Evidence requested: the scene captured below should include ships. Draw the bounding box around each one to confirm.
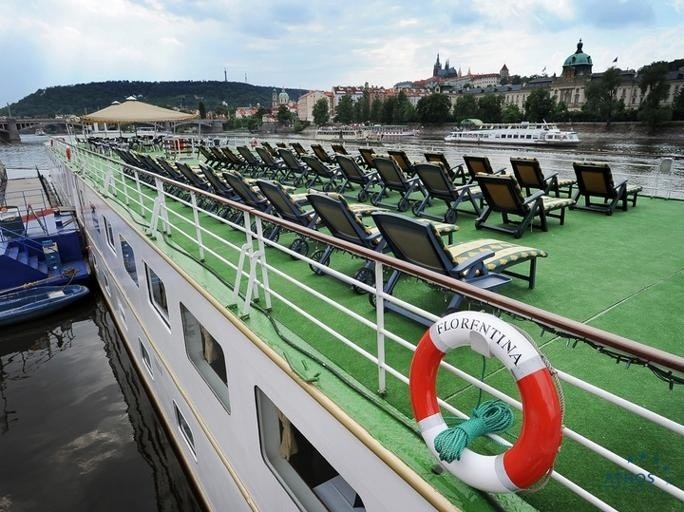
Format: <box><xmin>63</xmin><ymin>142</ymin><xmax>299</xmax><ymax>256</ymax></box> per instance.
<box><xmin>444</xmin><ymin>119</ymin><xmax>580</xmax><ymax>148</ymax></box>
<box><xmin>45</xmin><ymin>130</ymin><xmax>684</xmax><ymax>512</ymax></box>
<box><xmin>314</xmin><ymin>128</ymin><xmax>384</xmax><ymax>147</ymax></box>
<box><xmin>0</xmin><ymin>158</ymin><xmax>91</xmax><ymax>296</ymax></box>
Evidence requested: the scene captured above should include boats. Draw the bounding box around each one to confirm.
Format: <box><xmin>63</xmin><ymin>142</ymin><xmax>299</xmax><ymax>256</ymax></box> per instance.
<box><xmin>35</xmin><ymin>128</ymin><xmax>46</xmax><ymax>136</ymax></box>
<box><xmin>0</xmin><ymin>283</ymin><xmax>89</xmax><ymax>332</ymax></box>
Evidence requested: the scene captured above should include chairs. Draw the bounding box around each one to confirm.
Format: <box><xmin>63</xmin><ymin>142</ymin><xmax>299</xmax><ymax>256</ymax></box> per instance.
<box><xmin>300</xmin><ymin>153</ymin><xmax>355</xmax><ymax>192</ymax></box>
<box><xmin>358</xmin><ymin>148</ymin><xmax>376</xmax><ymax>171</ymax></box>
<box><xmin>113</xmin><ymin>148</ymin><xmax>202</xmax><ymax>191</ymax></box>
<box><xmin>306</xmin><ymin>188</ymin><xmax>459</xmax><ymax>295</ymax></box>
<box><xmin>254</xmin><ymin>179</ymin><xmax>391</xmax><ymax>261</ymax></box>
<box><xmin>209</xmin><ymin>146</ymin><xmax>237</xmax><ymax>170</ymax></box>
<box><xmin>568</xmin><ymin>162</ymin><xmax>643</xmax><ymax>216</ymax></box>
<box><xmin>195</xmin><ymin>146</ymin><xmax>223</xmax><ymax>167</ymax></box>
<box><xmin>331</xmin><ymin>144</ymin><xmax>365</xmax><ymax>169</ymax></box>
<box><xmin>221</xmin><ymin>168</ymin><xmax>312</xmax><ymax>240</ymax></box>
<box><xmin>510</xmin><ymin>157</ymin><xmax>576</xmax><ymax>198</ymax></box>
<box><xmin>236</xmin><ymin>145</ymin><xmax>276</xmax><ymax>178</ymax></box>
<box><xmin>198</xmin><ymin>161</ymin><xmax>297</xmax><ymax>223</ymax></box>
<box><xmin>74</xmin><ymin>133</ymin><xmax>230</xmax><ymax>160</ymax></box>
<box><xmin>311</xmin><ymin>144</ymin><xmax>338</xmax><ymax>166</ymax></box>
<box><xmin>292</xmin><ymin>143</ymin><xmax>314</xmax><ymax>159</ymax></box>
<box><xmin>412</xmin><ymin>161</ymin><xmax>488</xmax><ymax>224</ymax></box>
<box><xmin>261</xmin><ymin>141</ymin><xmax>277</xmax><ymax>156</ymax></box>
<box><xmin>255</xmin><ymin>147</ymin><xmax>296</xmax><ymax>180</ymax></box>
<box><xmin>220</xmin><ymin>147</ymin><xmax>255</xmax><ymax>171</ymax></box>
<box><xmin>463</xmin><ymin>154</ymin><xmax>507</xmax><ymax>181</ymax></box>
<box><xmin>370</xmin><ymin>154</ymin><xmax>433</xmax><ymax>212</ymax></box>
<box><xmin>424</xmin><ymin>152</ymin><xmax>474</xmax><ymax>186</ymax></box>
<box><xmin>155</xmin><ymin>156</ymin><xmax>222</xmax><ymax>202</ymax></box>
<box><xmin>278</xmin><ymin>149</ymin><xmax>324</xmax><ymax>188</ymax></box>
<box><xmin>387</xmin><ymin>150</ymin><xmax>419</xmax><ymax>178</ymax></box>
<box><xmin>335</xmin><ymin>156</ymin><xmax>390</xmax><ymax>202</ymax></box>
<box><xmin>369</xmin><ymin>212</ymin><xmax>548</xmax><ymax>318</ymax></box>
<box><xmin>175</xmin><ymin>162</ymin><xmax>257</xmax><ymax>212</ymax></box>
<box><xmin>475</xmin><ymin>172</ymin><xmax>577</xmax><ymax>239</ymax></box>
<box><xmin>276</xmin><ymin>143</ymin><xmax>286</xmax><ymax>160</ymax></box>
